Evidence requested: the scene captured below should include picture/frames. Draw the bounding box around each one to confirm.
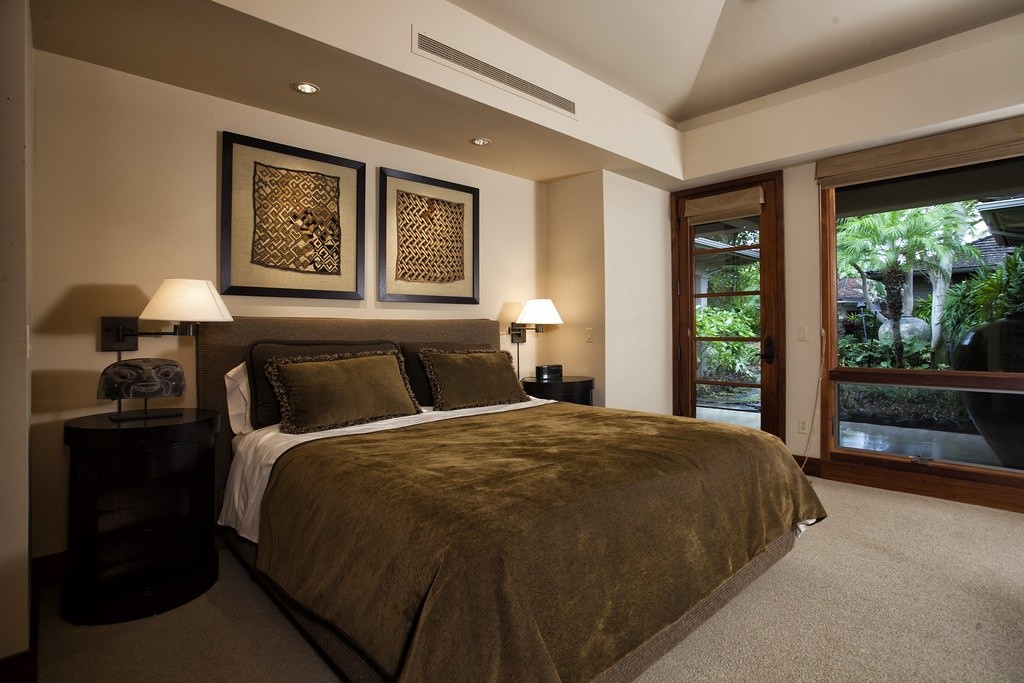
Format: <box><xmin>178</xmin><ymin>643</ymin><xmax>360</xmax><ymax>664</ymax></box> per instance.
<box><xmin>377</xmin><ymin>166</ymin><xmax>480</xmax><ymax>306</ymax></box>
<box><xmin>219</xmin><ymin>130</ymin><xmax>366</xmax><ymax>301</ymax></box>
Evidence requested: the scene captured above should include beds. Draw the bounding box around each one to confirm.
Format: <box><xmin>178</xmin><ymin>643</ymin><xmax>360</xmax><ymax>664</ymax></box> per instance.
<box><xmin>197</xmin><ymin>313</ymin><xmax>830</xmax><ymax>683</ymax></box>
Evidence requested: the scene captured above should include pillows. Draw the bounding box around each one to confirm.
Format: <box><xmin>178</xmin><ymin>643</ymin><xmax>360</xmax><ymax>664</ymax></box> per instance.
<box><xmin>396</xmin><ymin>340</ymin><xmax>498</xmax><ymax>407</ymax></box>
<box><xmin>416</xmin><ymin>346</ymin><xmax>532</xmax><ymax>412</ymax></box>
<box><xmin>264</xmin><ymin>348</ymin><xmax>423</xmax><ymax>435</ymax></box>
<box><xmin>245</xmin><ymin>337</ymin><xmax>401</xmax><ymax>431</ymax></box>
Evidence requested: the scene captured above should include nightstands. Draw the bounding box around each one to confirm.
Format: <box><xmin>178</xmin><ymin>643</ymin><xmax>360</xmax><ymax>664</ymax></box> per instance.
<box><xmin>60</xmin><ymin>409</ymin><xmax>223</xmax><ymax>627</ymax></box>
<box><xmin>522</xmin><ymin>376</ymin><xmax>595</xmax><ymax>406</ymax></box>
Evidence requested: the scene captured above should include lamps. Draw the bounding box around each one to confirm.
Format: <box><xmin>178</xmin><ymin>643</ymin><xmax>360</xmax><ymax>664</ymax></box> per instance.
<box><xmin>100</xmin><ymin>278</ymin><xmax>235</xmax><ymax>352</ymax></box>
<box><xmin>508</xmin><ymin>298</ymin><xmax>565</xmax><ymax>342</ymax></box>
